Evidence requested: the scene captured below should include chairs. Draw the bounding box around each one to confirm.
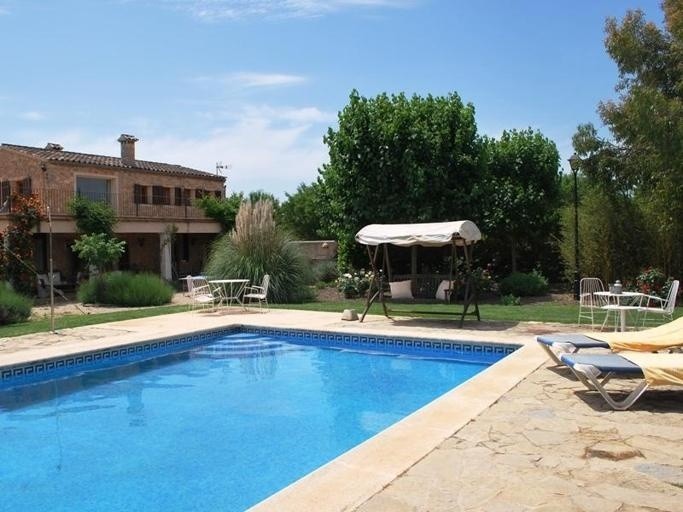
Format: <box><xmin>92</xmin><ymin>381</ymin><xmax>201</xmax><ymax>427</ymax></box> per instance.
<box><xmin>186</xmin><ymin>275</ymin><xmax>216</xmax><ymax>314</ymax></box>
<box><xmin>243</xmin><ymin>274</ymin><xmax>271</xmax><ymax>314</ymax></box>
<box><xmin>36</xmin><ymin>271</ymin><xmax>69</xmax><ymax>299</ymax></box>
<box><xmin>535</xmin><ymin>277</ymin><xmax>683</xmax><ymax>411</ymax></box>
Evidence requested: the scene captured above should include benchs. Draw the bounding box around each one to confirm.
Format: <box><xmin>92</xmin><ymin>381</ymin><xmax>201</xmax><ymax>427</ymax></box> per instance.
<box><xmin>360</xmin><ymin>273</ymin><xmax>480</xmax><ymax>329</ymax></box>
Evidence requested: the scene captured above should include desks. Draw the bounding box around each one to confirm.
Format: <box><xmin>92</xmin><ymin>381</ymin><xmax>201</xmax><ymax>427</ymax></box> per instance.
<box><xmin>207</xmin><ymin>278</ymin><xmax>250</xmax><ymax>312</ymax></box>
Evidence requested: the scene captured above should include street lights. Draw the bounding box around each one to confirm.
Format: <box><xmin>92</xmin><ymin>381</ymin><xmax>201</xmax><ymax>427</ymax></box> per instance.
<box><xmin>567</xmin><ymin>153</ymin><xmax>580</xmax><ymax>300</ymax></box>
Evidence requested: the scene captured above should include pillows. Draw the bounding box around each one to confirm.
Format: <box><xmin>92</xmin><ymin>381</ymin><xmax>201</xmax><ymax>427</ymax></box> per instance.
<box><xmin>435</xmin><ymin>279</ymin><xmax>455</xmax><ymax>302</ymax></box>
<box><xmin>388</xmin><ymin>279</ymin><xmax>415</xmax><ymax>300</ymax></box>
